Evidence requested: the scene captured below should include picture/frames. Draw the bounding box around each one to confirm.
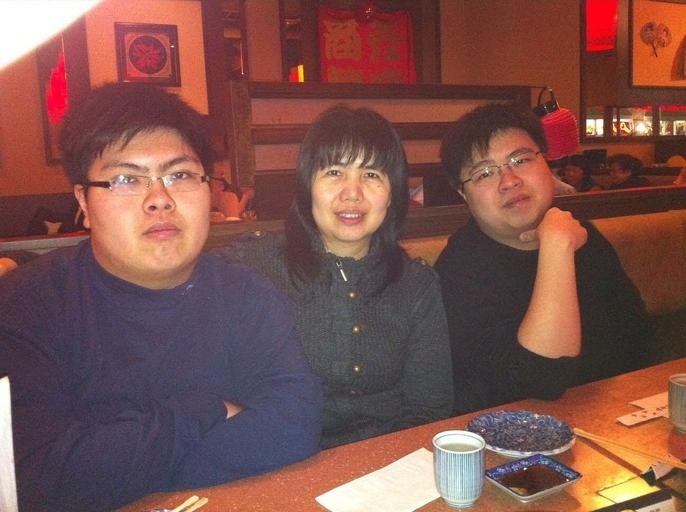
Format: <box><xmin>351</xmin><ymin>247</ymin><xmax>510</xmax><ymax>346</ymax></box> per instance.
<box><xmin>628</xmin><ymin>0</ymin><xmax>686</xmax><ymax>91</ymax></box>
<box><xmin>114</xmin><ymin>21</ymin><xmax>182</xmax><ymax>86</ymax></box>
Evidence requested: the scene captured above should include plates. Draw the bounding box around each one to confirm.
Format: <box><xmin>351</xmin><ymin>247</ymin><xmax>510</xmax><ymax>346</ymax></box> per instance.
<box><xmin>484</xmin><ymin>453</ymin><xmax>583</xmax><ymax>505</ymax></box>
<box><xmin>464</xmin><ymin>410</ymin><xmax>577</xmax><ymax>463</ymax></box>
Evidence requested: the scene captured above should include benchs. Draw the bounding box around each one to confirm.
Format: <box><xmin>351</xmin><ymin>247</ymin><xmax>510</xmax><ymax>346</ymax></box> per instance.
<box><xmin>589</xmin><ymin>166</ymin><xmax>681</xmax><ymax>189</ymax></box>
<box><xmin>15</xmin><ymin>185</ymin><xmax>685</xmax><ymax>365</ymax></box>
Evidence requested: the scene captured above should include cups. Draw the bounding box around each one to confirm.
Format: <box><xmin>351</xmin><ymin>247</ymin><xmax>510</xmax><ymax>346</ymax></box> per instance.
<box><xmin>668</xmin><ymin>373</ymin><xmax>686</xmax><ymax>437</ymax></box>
<box><xmin>432</xmin><ymin>430</ymin><xmax>485</xmax><ymax>509</ymax></box>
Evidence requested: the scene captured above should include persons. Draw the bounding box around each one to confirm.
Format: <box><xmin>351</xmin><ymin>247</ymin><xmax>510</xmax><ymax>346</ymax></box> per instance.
<box><xmin>1</xmin><ymin>80</ymin><xmax>324</xmax><ymax>512</ymax></box>
<box><xmin>556</xmin><ymin>153</ymin><xmax>603</xmax><ymax>194</ymax></box>
<box><xmin>433</xmin><ymin>102</ymin><xmax>656</xmax><ymax>417</ymax></box>
<box><xmin>222</xmin><ymin>100</ymin><xmax>456</xmax><ymax>451</ymax></box>
<box><xmin>607</xmin><ymin>154</ymin><xmax>651</xmax><ymax>190</ymax></box>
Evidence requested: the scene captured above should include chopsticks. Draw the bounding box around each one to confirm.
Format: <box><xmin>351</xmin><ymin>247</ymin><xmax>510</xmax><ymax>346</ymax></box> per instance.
<box><xmin>572</xmin><ymin>427</ymin><xmax>686</xmax><ymax>471</ymax></box>
<box><xmin>168</xmin><ymin>495</ymin><xmax>212</xmax><ymax>512</ymax></box>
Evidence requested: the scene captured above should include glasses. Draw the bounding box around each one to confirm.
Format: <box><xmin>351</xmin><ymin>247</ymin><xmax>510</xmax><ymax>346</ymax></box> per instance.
<box><xmin>84</xmin><ymin>172</ymin><xmax>209</xmax><ymax>196</ymax></box>
<box><xmin>461</xmin><ymin>149</ymin><xmax>542</xmax><ymax>192</ymax></box>
